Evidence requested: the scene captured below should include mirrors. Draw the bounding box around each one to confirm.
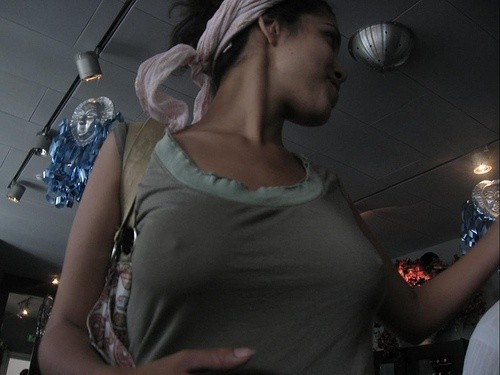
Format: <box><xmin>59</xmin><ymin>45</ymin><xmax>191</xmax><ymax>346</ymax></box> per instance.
<box><xmin>0</xmin><ymin>293</ymin><xmax>44</xmax><ymax>375</ymax></box>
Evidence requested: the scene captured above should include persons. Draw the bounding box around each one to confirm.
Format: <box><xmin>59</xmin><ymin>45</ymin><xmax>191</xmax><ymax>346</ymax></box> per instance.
<box><xmin>36</xmin><ymin>0</ymin><xmax>500</xmax><ymax>375</ymax></box>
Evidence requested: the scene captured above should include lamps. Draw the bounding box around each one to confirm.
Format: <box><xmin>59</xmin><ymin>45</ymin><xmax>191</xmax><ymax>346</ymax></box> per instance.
<box><xmin>471</xmin><ymin>145</ymin><xmax>493</xmax><ymax>175</ymax></box>
<box><xmin>16</xmin><ymin>305</ymin><xmax>29</xmax><ymax>320</ymax></box>
<box><xmin>31</xmin><ymin>135</ymin><xmax>51</xmax><ymax>156</ymax></box>
<box><xmin>7</xmin><ymin>184</ymin><xmax>26</xmax><ymax>203</ymax></box>
<box><xmin>73</xmin><ymin>51</ymin><xmax>102</xmax><ymax>81</ymax></box>
<box><xmin>346</xmin><ymin>20</ymin><xmax>417</xmax><ymax>72</ymax></box>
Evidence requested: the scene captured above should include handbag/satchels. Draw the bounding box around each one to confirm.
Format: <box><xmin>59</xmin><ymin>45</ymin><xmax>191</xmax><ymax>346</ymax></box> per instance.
<box><xmin>86</xmin><ymin>115</ymin><xmax>136</xmax><ymax>368</ymax></box>
